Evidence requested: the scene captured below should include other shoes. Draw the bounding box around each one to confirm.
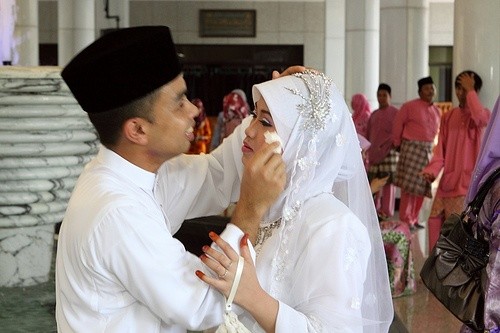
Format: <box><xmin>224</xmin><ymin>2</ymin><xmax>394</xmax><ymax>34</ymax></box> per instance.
<box><xmin>415</xmin><ymin>222</ymin><xmax>424</xmax><ymax>228</ymax></box>
<box><xmin>410</xmin><ymin>225</ymin><xmax>416</xmax><ymax>230</ymax></box>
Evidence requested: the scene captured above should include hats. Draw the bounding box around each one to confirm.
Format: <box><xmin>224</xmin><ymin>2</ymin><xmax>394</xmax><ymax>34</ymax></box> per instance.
<box><xmin>418</xmin><ymin>76</ymin><xmax>434</xmax><ymax>87</ymax></box>
<box><xmin>378</xmin><ymin>83</ymin><xmax>391</xmax><ymax>93</ymax></box>
<box><xmin>61</xmin><ymin>25</ymin><xmax>184</xmax><ymax>113</ymax></box>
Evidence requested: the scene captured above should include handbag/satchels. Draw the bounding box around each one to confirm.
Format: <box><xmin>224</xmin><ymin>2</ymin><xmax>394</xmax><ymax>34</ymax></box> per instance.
<box><xmin>214</xmin><ymin>256</ymin><xmax>252</xmax><ymax>333</ymax></box>
<box><xmin>420</xmin><ymin>167</ymin><xmax>500</xmax><ymax>333</ymax></box>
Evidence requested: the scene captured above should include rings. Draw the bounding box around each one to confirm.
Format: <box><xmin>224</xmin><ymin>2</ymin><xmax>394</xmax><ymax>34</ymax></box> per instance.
<box><xmin>219</xmin><ymin>270</ymin><xmax>227</xmax><ymax>279</ymax></box>
<box><xmin>228</xmin><ymin>262</ymin><xmax>233</xmax><ymax>270</ymax></box>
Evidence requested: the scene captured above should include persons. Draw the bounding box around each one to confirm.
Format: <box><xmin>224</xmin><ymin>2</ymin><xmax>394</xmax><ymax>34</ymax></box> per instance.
<box><xmin>362</xmin><ymin>83</ymin><xmax>403</xmax><ymax>225</ymax></box>
<box><xmin>55</xmin><ymin>25</ymin><xmax>308</xmax><ymax>333</ymax></box>
<box><xmin>459</xmin><ymin>95</ymin><xmax>500</xmax><ymax>333</ymax></box>
<box><xmin>349</xmin><ymin>94</ymin><xmax>371</xmax><ymax>173</ymax></box>
<box><xmin>422</xmin><ymin>70</ymin><xmax>491</xmax><ymax>256</ymax></box>
<box><xmin>392</xmin><ymin>76</ymin><xmax>442</xmax><ymax>232</ymax></box>
<box><xmin>195</xmin><ymin>69</ymin><xmax>395</xmax><ymax>333</ymax></box>
<box><xmin>186</xmin><ymin>89</ymin><xmax>250</xmax><ymax>156</ymax></box>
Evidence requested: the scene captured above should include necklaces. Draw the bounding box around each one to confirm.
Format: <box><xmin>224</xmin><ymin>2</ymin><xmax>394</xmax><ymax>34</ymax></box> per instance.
<box><xmin>252</xmin><ymin>216</ymin><xmax>282</xmax><ymax>247</ymax></box>
